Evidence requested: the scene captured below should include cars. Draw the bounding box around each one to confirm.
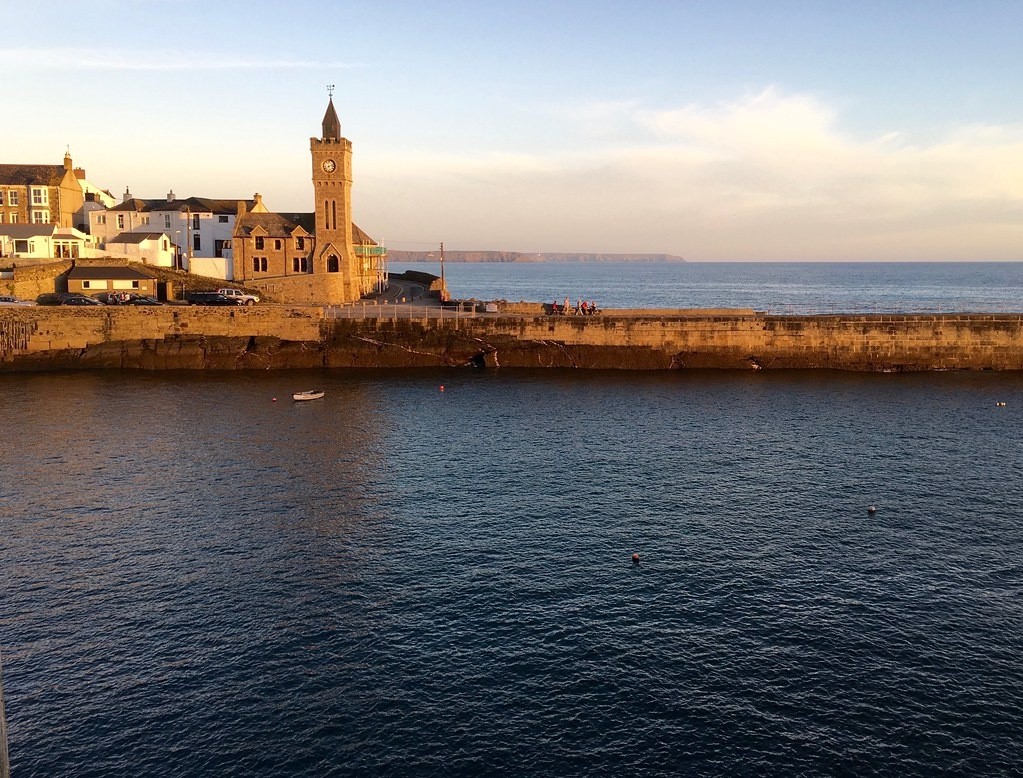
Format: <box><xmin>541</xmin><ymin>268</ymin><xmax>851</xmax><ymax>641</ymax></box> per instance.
<box><xmin>0</xmin><ymin>295</ymin><xmax>19</xmax><ymax>302</ymax></box>
<box><xmin>120</xmin><ymin>295</ymin><xmax>163</xmax><ymax>306</ymax></box>
<box><xmin>188</xmin><ymin>291</ymin><xmax>243</xmax><ymax>307</ymax></box>
<box><xmin>19</xmin><ymin>291</ymin><xmax>106</xmax><ymax>306</ymax></box>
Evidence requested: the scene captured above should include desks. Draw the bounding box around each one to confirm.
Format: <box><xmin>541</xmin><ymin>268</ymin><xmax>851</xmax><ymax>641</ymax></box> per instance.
<box><xmin>571</xmin><ymin>307</ymin><xmax>603</xmax><ymax>316</ymax></box>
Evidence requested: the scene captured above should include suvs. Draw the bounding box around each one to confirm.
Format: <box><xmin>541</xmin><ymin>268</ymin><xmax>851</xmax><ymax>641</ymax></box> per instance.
<box><xmin>218</xmin><ymin>288</ymin><xmax>260</xmax><ymax>306</ymax></box>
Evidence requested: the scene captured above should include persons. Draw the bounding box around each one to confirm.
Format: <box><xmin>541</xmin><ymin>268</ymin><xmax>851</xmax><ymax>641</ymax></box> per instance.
<box><xmin>218</xmin><ymin>290</ymin><xmax>224</xmax><ymax>297</ymax></box>
<box><xmin>552</xmin><ymin>301</ymin><xmax>559</xmax><ymax>315</ymax></box>
<box><xmin>121</xmin><ymin>291</ymin><xmax>125</xmax><ymax>300</ymax></box>
<box><xmin>125</xmin><ymin>293</ymin><xmax>130</xmax><ymax>301</ymax></box>
<box><xmin>105</xmin><ymin>292</ymin><xmax>110</xmax><ymax>304</ymax></box>
<box><xmin>131</xmin><ymin>291</ymin><xmax>138</xmax><ymax>300</ymax></box>
<box><xmin>563</xmin><ymin>297</ymin><xmax>570</xmax><ymax>316</ymax></box>
<box><xmin>589</xmin><ymin>301</ymin><xmax>597</xmax><ymax>315</ymax></box>
<box><xmin>112</xmin><ymin>292</ymin><xmax>121</xmax><ymax>305</ymax></box>
<box><xmin>581</xmin><ymin>300</ymin><xmax>590</xmax><ymax>315</ymax></box>
<box><xmin>576</xmin><ymin>297</ymin><xmax>584</xmax><ymax>316</ymax></box>
